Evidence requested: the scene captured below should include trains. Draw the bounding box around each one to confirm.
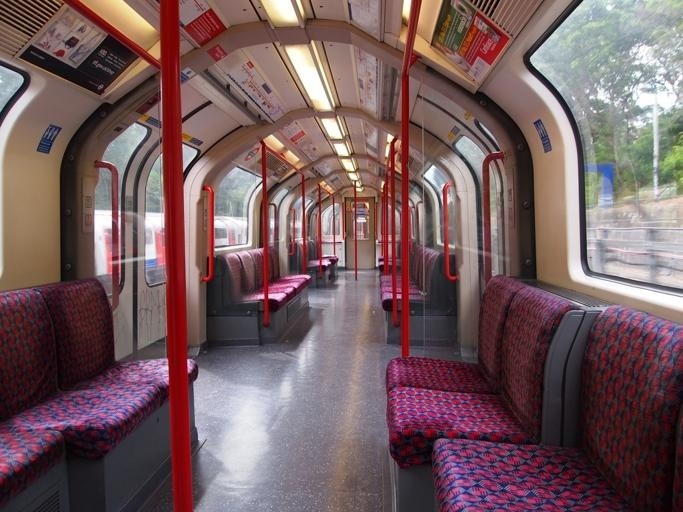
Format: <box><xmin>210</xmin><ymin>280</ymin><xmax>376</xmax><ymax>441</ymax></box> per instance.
<box><xmin>94</xmin><ymin>210</ymin><xmax>305</xmax><ymax>274</ymax></box>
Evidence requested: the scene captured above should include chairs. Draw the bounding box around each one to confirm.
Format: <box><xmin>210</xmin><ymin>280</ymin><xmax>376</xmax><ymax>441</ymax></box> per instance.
<box><xmin>0</xmin><ymin>276</ymin><xmax>201</xmax><ymax>511</ymax></box>
<box><xmin>377</xmin><ymin>237</ymin><xmax>682</xmax><ymax>510</ymax></box>
<box><xmin>202</xmin><ymin>237</ymin><xmax>340</xmax><ymax>348</ymax></box>
